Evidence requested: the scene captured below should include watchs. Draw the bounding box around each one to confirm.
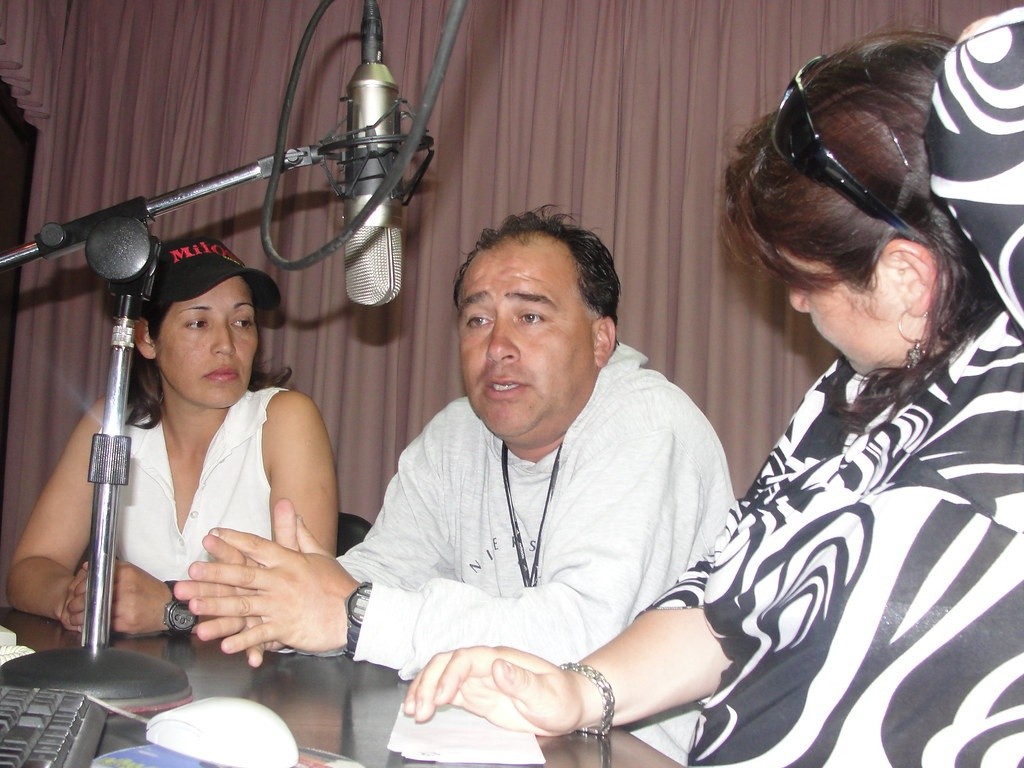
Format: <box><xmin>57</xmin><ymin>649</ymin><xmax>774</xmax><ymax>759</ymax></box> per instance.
<box><xmin>345</xmin><ymin>582</ymin><xmax>374</xmax><ymax>660</ymax></box>
<box><xmin>164</xmin><ymin>581</ymin><xmax>198</xmax><ymax>638</ymax></box>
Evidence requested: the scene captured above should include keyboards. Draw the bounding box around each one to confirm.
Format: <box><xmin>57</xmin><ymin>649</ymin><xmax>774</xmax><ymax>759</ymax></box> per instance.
<box><xmin>0</xmin><ymin>685</ymin><xmax>107</xmax><ymax>768</ymax></box>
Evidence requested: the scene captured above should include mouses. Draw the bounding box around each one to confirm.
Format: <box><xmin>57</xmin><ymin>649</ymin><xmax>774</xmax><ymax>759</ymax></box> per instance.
<box><xmin>145</xmin><ymin>697</ymin><xmax>300</xmax><ymax>768</ymax></box>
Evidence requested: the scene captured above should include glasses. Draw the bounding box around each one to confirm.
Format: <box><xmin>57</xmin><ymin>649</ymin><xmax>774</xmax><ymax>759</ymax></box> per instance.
<box><xmin>772</xmin><ymin>52</ymin><xmax>930</xmax><ymax>245</ymax></box>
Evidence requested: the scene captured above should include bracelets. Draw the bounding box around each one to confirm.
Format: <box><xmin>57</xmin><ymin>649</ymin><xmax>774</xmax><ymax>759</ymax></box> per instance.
<box><xmin>559</xmin><ymin>662</ymin><xmax>615</xmax><ymax>737</ymax></box>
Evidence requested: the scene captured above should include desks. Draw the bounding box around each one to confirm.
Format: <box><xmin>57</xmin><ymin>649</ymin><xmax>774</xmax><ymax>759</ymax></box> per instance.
<box><xmin>0</xmin><ymin>607</ymin><xmax>687</xmax><ymax>768</ymax></box>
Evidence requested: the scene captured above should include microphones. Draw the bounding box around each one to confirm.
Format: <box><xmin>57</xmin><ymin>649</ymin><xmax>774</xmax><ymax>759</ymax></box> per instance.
<box><xmin>345</xmin><ymin>0</ymin><xmax>402</xmax><ymax>307</ymax></box>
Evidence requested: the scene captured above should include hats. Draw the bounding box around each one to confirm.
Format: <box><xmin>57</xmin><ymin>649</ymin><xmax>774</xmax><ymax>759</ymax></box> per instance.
<box><xmin>147</xmin><ymin>236</ymin><xmax>281</xmax><ymax>311</ymax></box>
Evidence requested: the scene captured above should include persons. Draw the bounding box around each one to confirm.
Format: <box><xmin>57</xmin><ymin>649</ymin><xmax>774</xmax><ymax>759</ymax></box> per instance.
<box><xmin>6</xmin><ymin>232</ymin><xmax>339</xmax><ymax>636</ymax></box>
<box><xmin>174</xmin><ymin>202</ymin><xmax>738</xmax><ymax>767</ymax></box>
<box><xmin>405</xmin><ymin>5</ymin><xmax>1024</xmax><ymax>768</ymax></box>
